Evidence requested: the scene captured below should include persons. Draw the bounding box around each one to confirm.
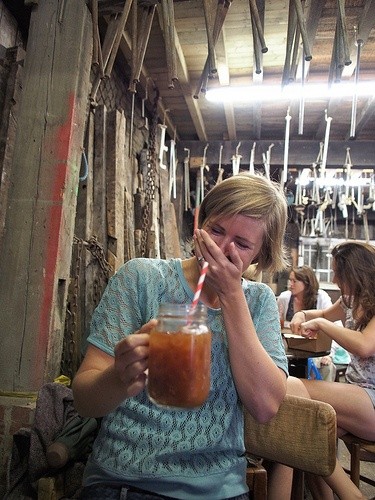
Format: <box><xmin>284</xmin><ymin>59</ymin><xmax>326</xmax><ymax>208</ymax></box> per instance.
<box><xmin>70</xmin><ymin>171</ymin><xmax>290</xmax><ymax>500</ymax></box>
<box><xmin>269</xmin><ymin>240</ymin><xmax>375</xmax><ymax>500</ymax></box>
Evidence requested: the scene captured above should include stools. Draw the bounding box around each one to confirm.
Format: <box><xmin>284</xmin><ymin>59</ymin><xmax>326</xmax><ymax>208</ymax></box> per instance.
<box><xmin>336</xmin><ymin>432</ymin><xmax>375</xmax><ymax>500</ymax></box>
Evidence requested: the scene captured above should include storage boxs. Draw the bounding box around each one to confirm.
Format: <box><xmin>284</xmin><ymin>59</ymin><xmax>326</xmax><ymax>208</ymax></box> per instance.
<box><xmin>284</xmin><ymin>331</ymin><xmax>332</xmax><ymax>353</ymax></box>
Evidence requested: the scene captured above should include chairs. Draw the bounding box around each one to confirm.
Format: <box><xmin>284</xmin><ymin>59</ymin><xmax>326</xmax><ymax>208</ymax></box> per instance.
<box><xmin>243</xmin><ymin>395</ymin><xmax>337</xmax><ymax>500</ymax></box>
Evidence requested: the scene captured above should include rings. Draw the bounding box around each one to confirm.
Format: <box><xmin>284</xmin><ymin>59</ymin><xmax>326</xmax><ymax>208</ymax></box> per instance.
<box><xmin>196</xmin><ymin>255</ymin><xmax>205</xmax><ymax>263</ymax></box>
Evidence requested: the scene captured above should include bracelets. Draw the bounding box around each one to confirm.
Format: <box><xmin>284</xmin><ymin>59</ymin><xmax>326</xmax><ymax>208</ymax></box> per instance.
<box><xmin>301</xmin><ymin>310</ymin><xmax>306</xmax><ymax>316</ymax></box>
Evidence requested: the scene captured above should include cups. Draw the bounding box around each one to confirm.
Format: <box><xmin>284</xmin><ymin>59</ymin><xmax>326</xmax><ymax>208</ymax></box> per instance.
<box><xmin>138</xmin><ymin>303</ymin><xmax>211</xmax><ymax>409</ymax></box>
